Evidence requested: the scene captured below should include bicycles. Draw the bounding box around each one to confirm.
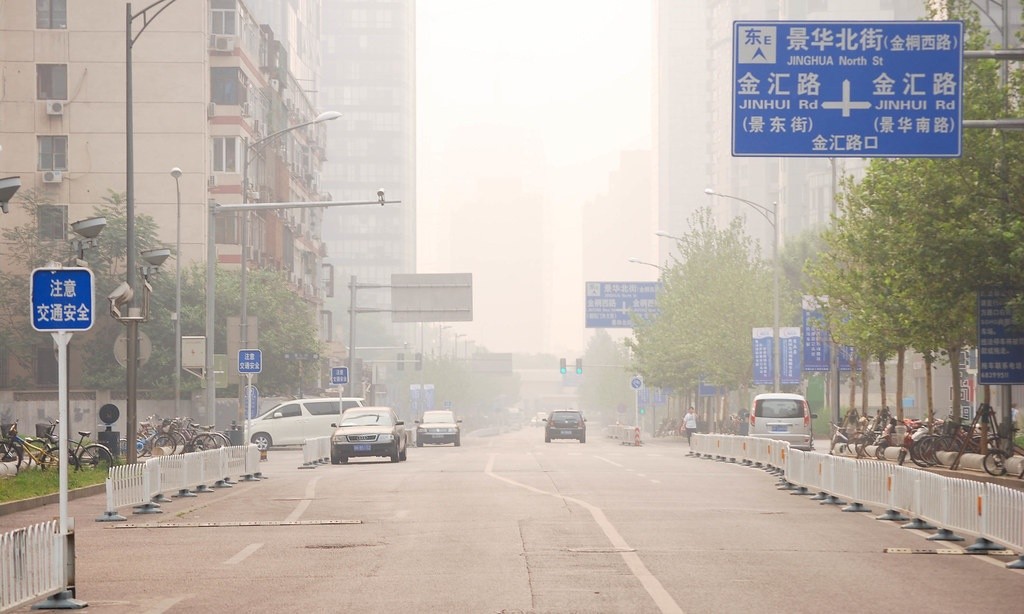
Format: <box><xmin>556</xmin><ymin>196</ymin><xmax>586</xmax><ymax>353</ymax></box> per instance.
<box><xmin>120</xmin><ymin>414</ymin><xmax>234</xmax><ymax>459</ymax></box>
<box><xmin>828</xmin><ymin>414</ymin><xmax>1024</xmax><ymax>477</ymax></box>
<box><xmin>655</xmin><ymin>413</ymin><xmax>741</xmax><ymax>437</ymax></box>
<box><xmin>0</xmin><ymin>418</ymin><xmax>114</xmax><ymax>475</ymax></box>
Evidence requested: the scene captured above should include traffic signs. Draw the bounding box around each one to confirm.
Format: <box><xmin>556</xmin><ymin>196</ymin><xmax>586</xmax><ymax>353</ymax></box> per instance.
<box><xmin>584</xmin><ymin>281</ymin><xmax>666</xmax><ymax>329</ymax></box>
<box><xmin>732</xmin><ymin>20</ymin><xmax>963</xmax><ymax>159</ymax></box>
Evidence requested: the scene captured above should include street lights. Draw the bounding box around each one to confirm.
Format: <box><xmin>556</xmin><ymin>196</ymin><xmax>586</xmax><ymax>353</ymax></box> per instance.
<box><xmin>439</xmin><ymin>325</ymin><xmax>452</xmax><ymax>358</ymax></box>
<box><xmin>704</xmin><ymin>189</ymin><xmax>780</xmax><ymax>394</ymax></box>
<box><xmin>455</xmin><ymin>333</ymin><xmax>475</xmax><ymax>359</ymax></box>
<box><xmin>172</xmin><ymin>167</ymin><xmax>184</xmax><ymax>420</ymax></box>
<box><xmin>238</xmin><ymin>111</ymin><xmax>342</xmax><ymax>415</ymax></box>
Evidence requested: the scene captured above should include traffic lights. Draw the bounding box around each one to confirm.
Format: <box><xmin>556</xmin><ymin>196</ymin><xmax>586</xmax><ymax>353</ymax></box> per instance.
<box><xmin>560</xmin><ymin>358</ymin><xmax>567</xmax><ymax>374</ymax></box>
<box><xmin>576</xmin><ymin>358</ymin><xmax>582</xmax><ymax>374</ymax></box>
<box><xmin>640</xmin><ymin>408</ymin><xmax>645</xmax><ymax>415</ymax></box>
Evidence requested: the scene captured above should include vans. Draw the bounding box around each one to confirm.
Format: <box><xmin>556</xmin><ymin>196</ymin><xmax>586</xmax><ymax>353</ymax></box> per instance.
<box><xmin>743</xmin><ymin>393</ymin><xmax>818</xmax><ymax>452</ymax></box>
<box><xmin>243</xmin><ymin>398</ymin><xmax>367</xmax><ymax>452</ymax></box>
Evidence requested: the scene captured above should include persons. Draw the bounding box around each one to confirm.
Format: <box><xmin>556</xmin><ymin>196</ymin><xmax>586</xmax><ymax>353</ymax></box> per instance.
<box><xmin>1011</xmin><ymin>402</ymin><xmax>1018</xmax><ymax>428</ymax></box>
<box><xmin>684</xmin><ymin>407</ymin><xmax>696</xmax><ymax>445</ymax></box>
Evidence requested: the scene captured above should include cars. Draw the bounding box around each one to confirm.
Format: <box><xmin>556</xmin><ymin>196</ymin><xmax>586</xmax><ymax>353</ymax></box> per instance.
<box><xmin>415</xmin><ymin>410</ymin><xmax>463</xmax><ymax>447</ymax></box>
<box><xmin>530</xmin><ymin>412</ymin><xmax>549</xmax><ymax>427</ymax></box>
<box><xmin>330</xmin><ymin>406</ymin><xmax>408</xmax><ymax>465</ymax></box>
<box><xmin>542</xmin><ymin>410</ymin><xmax>587</xmax><ymax>443</ymax></box>
